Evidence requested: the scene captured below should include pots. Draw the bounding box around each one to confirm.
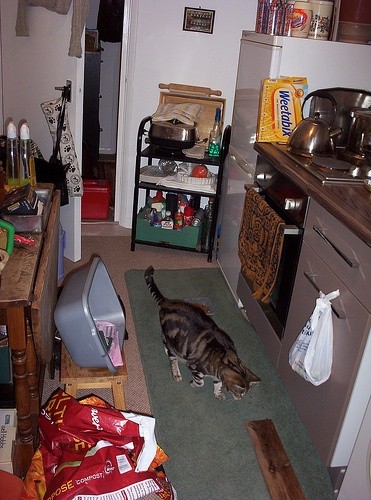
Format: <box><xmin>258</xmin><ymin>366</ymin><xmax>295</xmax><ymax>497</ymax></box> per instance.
<box><xmin>349</xmin><ymin>108</ymin><xmax>371</xmax><ymax>156</ymax></box>
<box><xmin>142</xmin><ymin>118</ymin><xmax>209</xmax><ymax>155</ymax></box>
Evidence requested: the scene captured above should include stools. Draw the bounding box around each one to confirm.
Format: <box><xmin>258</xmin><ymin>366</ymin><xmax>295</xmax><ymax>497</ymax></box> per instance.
<box><xmin>60</xmin><ymin>339</ymin><xmax>129</xmax><ymax>413</ymax></box>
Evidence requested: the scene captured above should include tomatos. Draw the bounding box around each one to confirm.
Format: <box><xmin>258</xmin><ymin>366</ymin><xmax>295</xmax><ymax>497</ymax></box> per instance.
<box><xmin>193</xmin><ymin>165</ymin><xmax>208</xmax><ymax>179</ymax></box>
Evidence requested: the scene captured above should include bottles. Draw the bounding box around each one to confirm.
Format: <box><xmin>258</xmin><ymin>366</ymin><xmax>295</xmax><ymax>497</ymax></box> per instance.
<box><xmin>19</xmin><ymin>123</ymin><xmax>37</xmax><ymax>186</ymax></box>
<box><xmin>208</xmin><ymin>108</ymin><xmax>222</xmax><ymax>158</ymax></box>
<box><xmin>161</xmin><ymin>192</ymin><xmax>204</xmax><ymax>230</ymax></box>
<box><xmin>6</xmin><ymin>121</ymin><xmax>19</xmax><ymax>186</ymax></box>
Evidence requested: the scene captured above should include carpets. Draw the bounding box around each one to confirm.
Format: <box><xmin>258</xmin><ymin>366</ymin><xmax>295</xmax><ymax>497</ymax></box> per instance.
<box><xmin>125</xmin><ymin>267</ymin><xmax>336</xmax><ymax>500</ymax></box>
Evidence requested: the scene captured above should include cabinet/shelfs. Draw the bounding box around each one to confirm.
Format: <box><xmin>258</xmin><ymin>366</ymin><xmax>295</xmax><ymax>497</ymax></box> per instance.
<box><xmin>276</xmin><ymin>198</ymin><xmax>371</xmax><ymax>498</ymax></box>
<box><xmin>130</xmin><ymin>116</ymin><xmax>232</xmax><ymax>263</ymax></box>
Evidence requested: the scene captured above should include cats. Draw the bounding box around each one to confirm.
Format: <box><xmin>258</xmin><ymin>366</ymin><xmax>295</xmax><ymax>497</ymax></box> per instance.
<box><xmin>144</xmin><ymin>265</ymin><xmax>262</xmax><ymax>401</ymax></box>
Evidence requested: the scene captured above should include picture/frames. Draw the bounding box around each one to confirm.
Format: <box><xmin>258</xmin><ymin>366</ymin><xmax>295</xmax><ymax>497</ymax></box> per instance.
<box><xmin>183</xmin><ymin>7</ymin><xmax>215</xmax><ymax>34</ymax></box>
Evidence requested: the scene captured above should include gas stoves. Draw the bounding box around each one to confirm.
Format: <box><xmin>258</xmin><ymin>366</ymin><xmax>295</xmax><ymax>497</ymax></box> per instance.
<box><xmin>271</xmin><ymin>140</ymin><xmax>371</xmax><ymax>187</ymax></box>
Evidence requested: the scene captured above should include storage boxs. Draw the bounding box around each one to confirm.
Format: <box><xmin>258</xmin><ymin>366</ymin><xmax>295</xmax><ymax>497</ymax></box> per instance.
<box><xmin>135</xmin><ymin>202</ymin><xmax>204</xmax><ymax>248</ymax></box>
<box><xmin>256</xmin><ymin>76</ymin><xmax>308</xmax><ymax>143</ymax></box>
<box><xmin>54</xmin><ymin>256</ymin><xmax>125</xmax><ymax>373</ymax></box>
<box><xmin>0</xmin><ymin>334</ymin><xmax>14</xmax><ymax>385</ymax></box>
<box><xmin>82</xmin><ymin>180</ymin><xmax>112</xmax><ymax>219</ymax></box>
<box><xmin>0</xmin><ymin>409</ymin><xmax>17</xmax><ymax>475</ymax></box>
<box><xmin>0</xmin><ymin>183</ymin><xmax>51</xmax><ymax>233</ymax></box>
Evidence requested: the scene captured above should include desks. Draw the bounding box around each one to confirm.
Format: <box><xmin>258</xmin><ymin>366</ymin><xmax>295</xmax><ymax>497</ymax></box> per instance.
<box><xmin>0</xmin><ymin>182</ymin><xmax>61</xmax><ymax>479</ymax></box>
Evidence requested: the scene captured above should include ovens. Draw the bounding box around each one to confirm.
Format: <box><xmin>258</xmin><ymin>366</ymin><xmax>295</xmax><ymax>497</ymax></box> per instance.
<box><xmin>240</xmin><ymin>155</ymin><xmax>311</xmax><ymax>342</ymax></box>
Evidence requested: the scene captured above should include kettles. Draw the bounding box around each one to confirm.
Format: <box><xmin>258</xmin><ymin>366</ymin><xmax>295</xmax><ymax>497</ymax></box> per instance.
<box><xmin>287</xmin><ymin>92</ymin><xmax>343</xmax><ymax>158</ymax></box>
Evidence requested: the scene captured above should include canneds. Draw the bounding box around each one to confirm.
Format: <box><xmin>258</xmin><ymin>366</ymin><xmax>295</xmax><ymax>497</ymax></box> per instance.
<box><xmin>290</xmin><ymin>1</ymin><xmax>313</xmax><ymax>38</ymax></box>
